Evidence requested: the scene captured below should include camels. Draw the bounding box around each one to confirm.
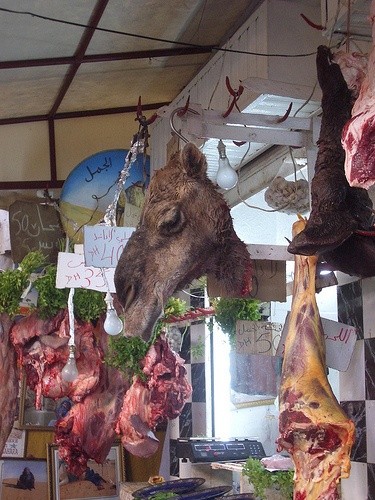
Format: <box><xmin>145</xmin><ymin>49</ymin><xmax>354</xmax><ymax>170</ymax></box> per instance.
<box><xmin>112</xmin><ymin>143</ymin><xmax>252</xmax><ymax>342</ymax></box>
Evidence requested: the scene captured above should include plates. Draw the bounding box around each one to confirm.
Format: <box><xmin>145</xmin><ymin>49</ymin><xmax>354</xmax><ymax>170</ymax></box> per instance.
<box><xmin>131</xmin><ymin>478</ymin><xmax>205</xmax><ymax>500</ymax></box>
<box><xmin>178</xmin><ymin>486</ymin><xmax>233</xmax><ymax>500</ymax></box>
<box><xmin>207</xmin><ymin>493</ymin><xmax>259</xmax><ymax>500</ymax></box>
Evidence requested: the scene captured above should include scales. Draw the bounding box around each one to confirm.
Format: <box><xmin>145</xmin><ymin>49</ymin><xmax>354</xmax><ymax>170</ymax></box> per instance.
<box><xmin>170</xmin><ymin>436</ymin><xmax>267</xmax><ymax>463</ymax></box>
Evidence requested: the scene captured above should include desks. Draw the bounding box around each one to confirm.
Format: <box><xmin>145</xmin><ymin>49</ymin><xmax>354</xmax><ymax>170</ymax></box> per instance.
<box><xmin>120</xmin><ymin>479</ymin><xmax>179</xmax><ymax>500</ymax></box>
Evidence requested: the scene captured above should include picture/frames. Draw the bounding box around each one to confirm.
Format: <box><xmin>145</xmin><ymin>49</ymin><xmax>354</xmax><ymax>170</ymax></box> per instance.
<box><xmin>45</xmin><ymin>438</ymin><xmax>125</xmax><ymax>500</ymax></box>
